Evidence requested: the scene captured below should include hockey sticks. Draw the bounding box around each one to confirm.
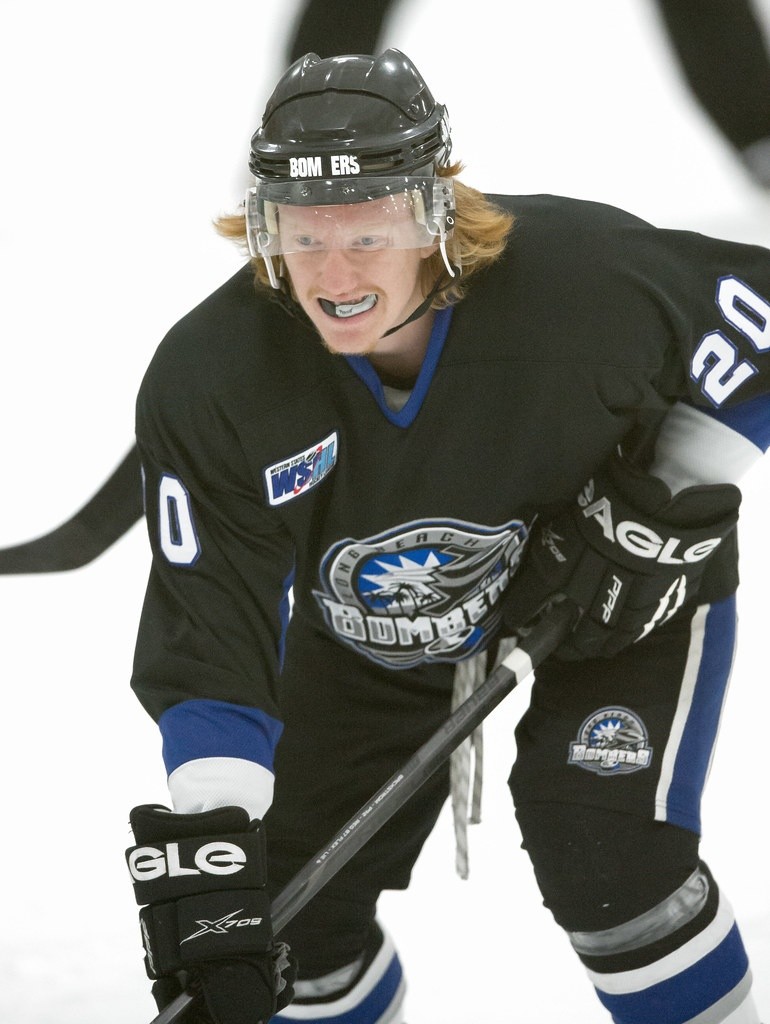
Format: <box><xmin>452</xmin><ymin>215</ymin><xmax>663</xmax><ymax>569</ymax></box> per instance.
<box><xmin>143</xmin><ymin>597</ymin><xmax>585</xmax><ymax>1024</ymax></box>
<box><xmin>0</xmin><ymin>441</ymin><xmax>146</xmax><ymax>577</ymax></box>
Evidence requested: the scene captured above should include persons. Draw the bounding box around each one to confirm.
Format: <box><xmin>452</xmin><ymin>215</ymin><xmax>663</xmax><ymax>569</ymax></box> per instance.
<box><xmin>123</xmin><ymin>48</ymin><xmax>768</xmax><ymax>1024</ymax></box>
<box><xmin>289</xmin><ymin>0</ymin><xmax>770</xmax><ymax>193</ymax></box>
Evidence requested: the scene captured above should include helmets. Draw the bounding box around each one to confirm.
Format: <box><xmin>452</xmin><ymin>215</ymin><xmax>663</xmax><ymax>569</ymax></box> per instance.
<box><xmin>243</xmin><ymin>47</ymin><xmax>455</xmax><ymax>290</ymax></box>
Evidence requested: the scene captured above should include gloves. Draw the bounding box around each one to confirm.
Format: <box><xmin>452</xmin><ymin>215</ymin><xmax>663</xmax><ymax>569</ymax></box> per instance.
<box><xmin>125</xmin><ymin>804</ymin><xmax>298</xmax><ymax>1024</ymax></box>
<box><xmin>497</xmin><ymin>455</ymin><xmax>742</xmax><ymax>669</ymax></box>
